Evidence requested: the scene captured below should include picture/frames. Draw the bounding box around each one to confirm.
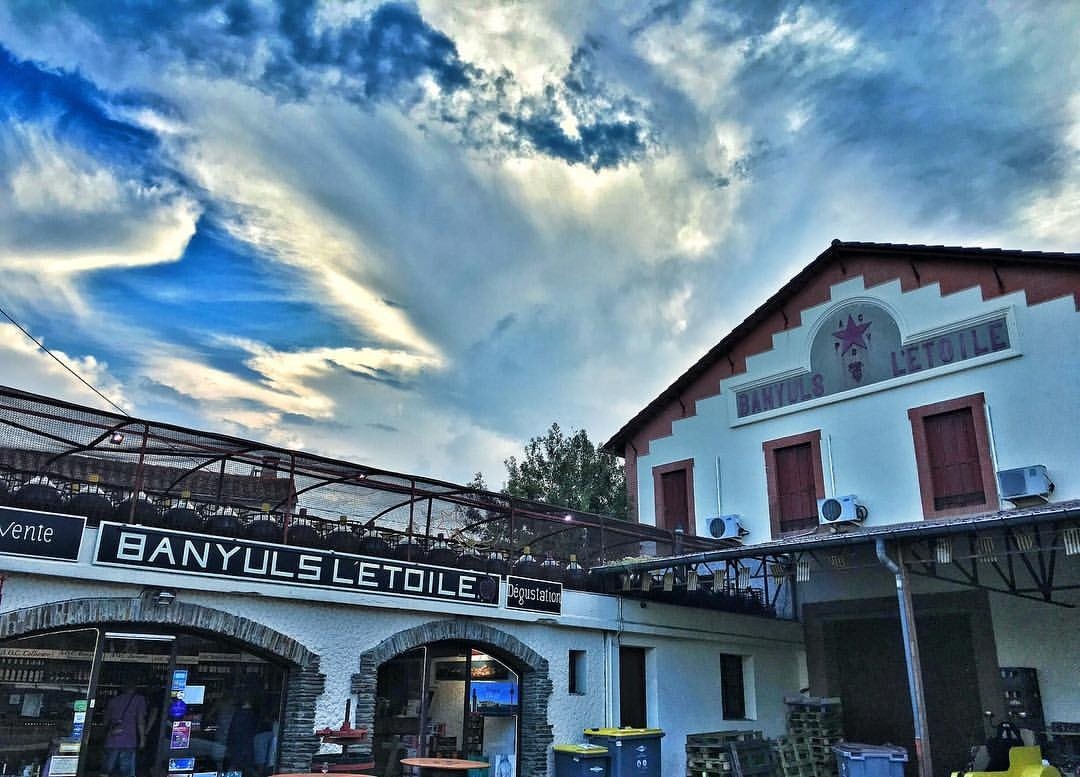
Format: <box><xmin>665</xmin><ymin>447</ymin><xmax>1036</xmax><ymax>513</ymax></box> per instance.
<box><xmin>436</xmin><ymin>659</ymin><xmax>510</xmax><ymax>680</ymax></box>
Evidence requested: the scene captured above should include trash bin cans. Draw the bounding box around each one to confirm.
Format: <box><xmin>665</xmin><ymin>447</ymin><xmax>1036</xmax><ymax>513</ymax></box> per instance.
<box><xmin>831</xmin><ymin>742</ymin><xmax>909</xmax><ymax>777</ymax></box>
<box><xmin>553</xmin><ymin>743</ymin><xmax>609</xmax><ymax>777</ymax></box>
<box><xmin>583</xmin><ymin>725</ymin><xmax>666</xmax><ymax>777</ymax></box>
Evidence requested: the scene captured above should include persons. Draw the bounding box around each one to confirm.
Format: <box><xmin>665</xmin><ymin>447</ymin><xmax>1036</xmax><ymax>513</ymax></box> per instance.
<box><xmin>141</xmin><ymin>686</ymin><xmax>166</xmax><ymax>777</ymax></box>
<box><xmin>99</xmin><ymin>677</ymin><xmax>147</xmax><ymax>777</ymax></box>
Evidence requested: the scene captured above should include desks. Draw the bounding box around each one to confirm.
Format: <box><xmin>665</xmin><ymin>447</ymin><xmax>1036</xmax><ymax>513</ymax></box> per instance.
<box><xmin>401</xmin><ymin>757</ymin><xmax>490</xmax><ymax>777</ymax></box>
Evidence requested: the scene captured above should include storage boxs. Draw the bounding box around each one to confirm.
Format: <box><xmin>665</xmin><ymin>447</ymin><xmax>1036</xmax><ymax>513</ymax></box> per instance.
<box><xmin>999</xmin><ymin>666</ymin><xmax>1080</xmax><ymax>768</ymax></box>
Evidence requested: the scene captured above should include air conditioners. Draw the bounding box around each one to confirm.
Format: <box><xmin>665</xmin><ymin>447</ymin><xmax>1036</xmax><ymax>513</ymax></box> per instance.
<box><xmin>706</xmin><ymin>515</ymin><xmax>742</xmax><ymax>539</ymax></box>
<box><xmin>817</xmin><ymin>495</ymin><xmax>859</xmax><ymax>523</ymax></box>
<box><xmin>996</xmin><ymin>465</ymin><xmax>1049</xmax><ymax>496</ymax></box>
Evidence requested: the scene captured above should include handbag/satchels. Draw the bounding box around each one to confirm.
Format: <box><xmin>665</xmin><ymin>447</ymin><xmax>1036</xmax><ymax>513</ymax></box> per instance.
<box><xmin>109</xmin><ymin>719</ymin><xmax>123</xmax><ymax>736</ymax></box>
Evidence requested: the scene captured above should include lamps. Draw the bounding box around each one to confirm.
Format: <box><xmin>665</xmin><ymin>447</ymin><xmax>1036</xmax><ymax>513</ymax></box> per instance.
<box><xmin>104</xmin><ymin>632</ymin><xmax>176</xmax><ymax>642</ymax></box>
<box><xmin>154</xmin><ymin>589</ymin><xmax>176</xmax><ymax>608</ymax></box>
